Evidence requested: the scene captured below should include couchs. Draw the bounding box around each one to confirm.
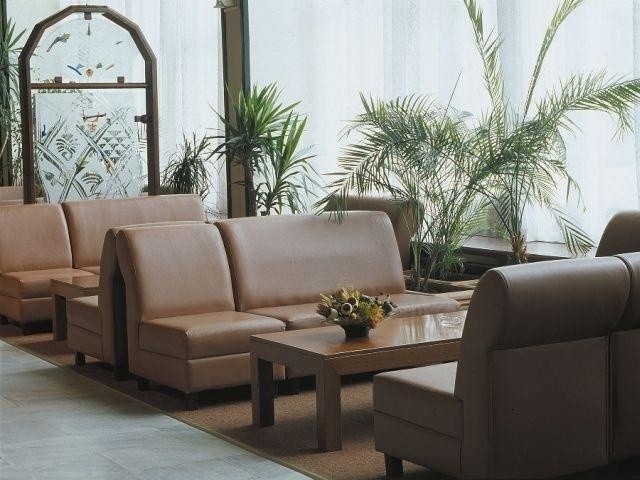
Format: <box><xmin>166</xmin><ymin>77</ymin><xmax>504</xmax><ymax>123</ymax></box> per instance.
<box><xmin>368</xmin><ymin>207</ymin><xmax>640</xmax><ymax>480</ymax></box>
<box><xmin>328</xmin><ymin>195</ymin><xmax>425</xmax><ymax>290</ymax></box>
<box><xmin>115</xmin><ymin>210</ymin><xmax>462</xmax><ymax>411</ymax></box>
<box><xmin>2</xmin><ymin>185</ymin><xmax>43</xmax><ymax>207</ymax></box>
<box><xmin>67</xmin><ymin>219</ymin><xmax>214</xmax><ymax>373</ymax></box>
<box><xmin>4</xmin><ymin>194</ymin><xmax>208</xmax><ymax>336</ymax></box>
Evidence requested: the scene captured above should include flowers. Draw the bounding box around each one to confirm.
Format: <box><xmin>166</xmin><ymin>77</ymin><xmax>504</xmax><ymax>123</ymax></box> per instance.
<box><xmin>315</xmin><ymin>289</ymin><xmax>399</xmax><ymax>320</ymax></box>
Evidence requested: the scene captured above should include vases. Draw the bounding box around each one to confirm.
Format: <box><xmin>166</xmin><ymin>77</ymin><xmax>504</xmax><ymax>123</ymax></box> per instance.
<box><xmin>334</xmin><ymin>319</ymin><xmax>379</xmax><ymax>340</ymax></box>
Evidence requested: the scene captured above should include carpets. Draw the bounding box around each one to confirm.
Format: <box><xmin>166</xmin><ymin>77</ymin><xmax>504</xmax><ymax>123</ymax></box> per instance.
<box><xmin>1</xmin><ymin>321</ymin><xmax>640</xmax><ymax>480</ymax></box>
